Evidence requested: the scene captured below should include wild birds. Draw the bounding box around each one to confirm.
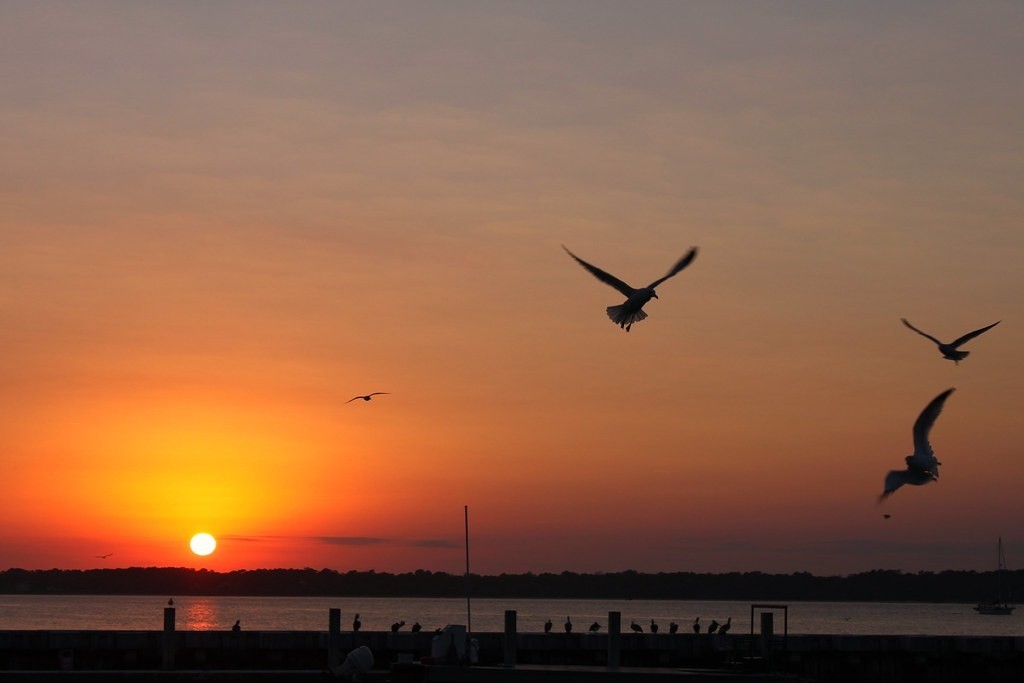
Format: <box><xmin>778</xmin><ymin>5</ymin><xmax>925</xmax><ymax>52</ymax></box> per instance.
<box><xmin>629</xmin><ymin>617</ymin><xmax>732</xmax><ymax>634</ymax></box>
<box><xmin>897</xmin><ymin>315</ymin><xmax>1002</xmax><ymax>367</ymax></box>
<box><xmin>352</xmin><ymin>612</ymin><xmax>423</xmax><ymax>632</ymax></box>
<box><xmin>92</xmin><ymin>552</ymin><xmax>115</xmax><ymax>560</ymax></box>
<box><xmin>343</xmin><ymin>391</ymin><xmax>392</xmax><ymax>406</ymax></box>
<box><xmin>542</xmin><ymin>615</ymin><xmax>603</xmax><ymax>632</ymax></box>
<box><xmin>876</xmin><ymin>387</ymin><xmax>959</xmax><ymax>518</ymax></box>
<box><xmin>229</xmin><ymin>618</ymin><xmax>241</xmax><ymax>632</ymax></box>
<box><xmin>167</xmin><ymin>596</ymin><xmax>175</xmax><ymax>609</ymax></box>
<box><xmin>557</xmin><ymin>240</ymin><xmax>700</xmax><ymax>335</ymax></box>
